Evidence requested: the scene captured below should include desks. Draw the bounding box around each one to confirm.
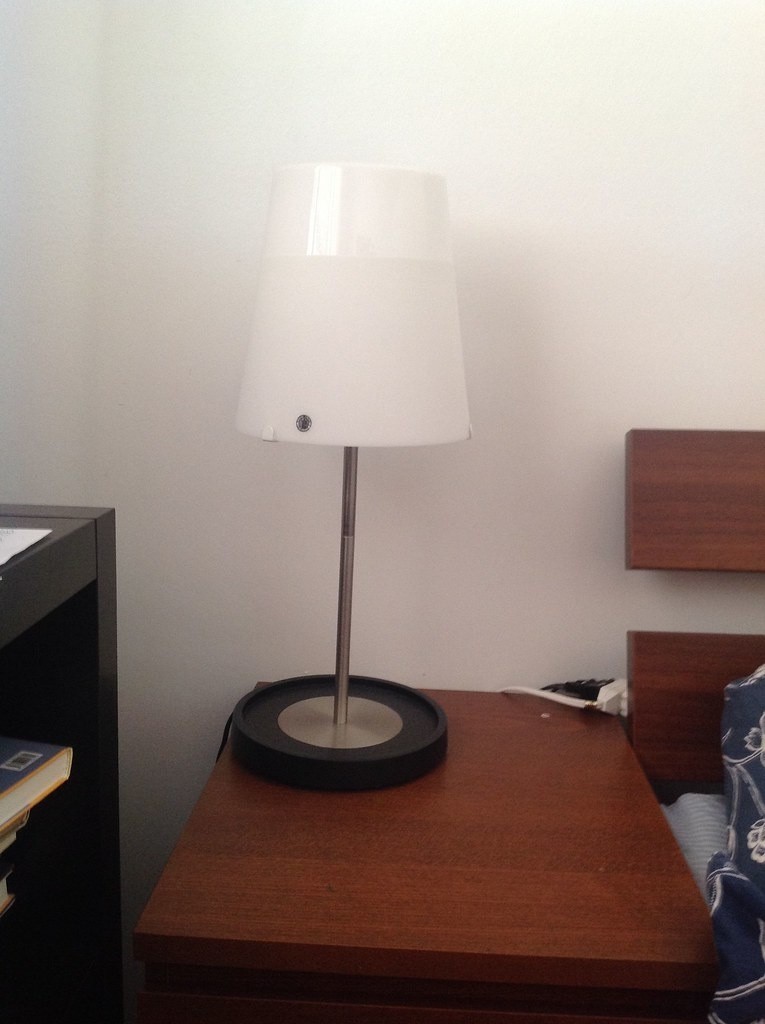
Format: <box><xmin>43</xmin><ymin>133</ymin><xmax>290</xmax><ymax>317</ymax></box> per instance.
<box><xmin>0</xmin><ymin>502</ymin><xmax>124</xmax><ymax>1024</ymax></box>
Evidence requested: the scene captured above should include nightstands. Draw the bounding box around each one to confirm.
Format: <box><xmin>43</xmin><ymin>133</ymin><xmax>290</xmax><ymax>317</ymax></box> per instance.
<box><xmin>133</xmin><ymin>681</ymin><xmax>720</xmax><ymax>1024</ymax></box>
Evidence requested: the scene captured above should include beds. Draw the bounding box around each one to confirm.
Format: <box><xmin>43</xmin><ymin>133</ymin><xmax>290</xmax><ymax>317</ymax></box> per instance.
<box><xmin>625</xmin><ymin>424</ymin><xmax>765</xmax><ymax>1024</ymax></box>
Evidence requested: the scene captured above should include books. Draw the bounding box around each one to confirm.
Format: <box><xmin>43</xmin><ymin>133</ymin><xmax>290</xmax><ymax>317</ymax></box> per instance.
<box><xmin>0</xmin><ymin>734</ymin><xmax>74</xmax><ymax>929</ymax></box>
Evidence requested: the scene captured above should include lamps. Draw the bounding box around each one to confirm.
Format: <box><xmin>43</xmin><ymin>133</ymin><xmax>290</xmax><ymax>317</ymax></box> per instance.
<box><xmin>230</xmin><ymin>162</ymin><xmax>471</xmax><ymax>793</ymax></box>
